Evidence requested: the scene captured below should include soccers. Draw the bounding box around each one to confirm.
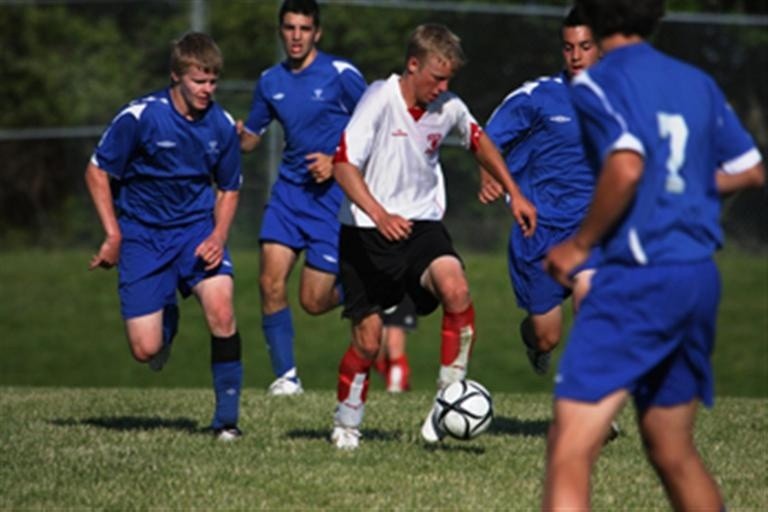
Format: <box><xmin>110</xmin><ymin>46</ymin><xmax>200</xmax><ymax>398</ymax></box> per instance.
<box><xmin>435</xmin><ymin>379</ymin><xmax>497</xmax><ymax>436</ymax></box>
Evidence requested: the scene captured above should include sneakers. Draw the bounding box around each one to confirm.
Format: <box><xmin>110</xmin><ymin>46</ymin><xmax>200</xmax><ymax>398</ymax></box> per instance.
<box><xmin>148</xmin><ymin>303</ymin><xmax>180</xmax><ymax>373</ymax></box>
<box><xmin>265</xmin><ymin>374</ymin><xmax>305</xmax><ymax>399</ymax></box>
<box><xmin>373</xmin><ymin>351</ymin><xmax>411</xmax><ymax>393</ymax></box>
<box><xmin>519</xmin><ymin>317</ymin><xmax>553</xmax><ymax>377</ymax></box>
<box><xmin>211</xmin><ymin>419</ymin><xmax>243</xmax><ymax>442</ymax></box>
<box><xmin>330</xmin><ymin>405</ymin><xmax>363</xmax><ymax>451</ymax></box>
<box><xmin>420</xmin><ymin>379</ymin><xmax>448</xmax><ymax>446</ymax></box>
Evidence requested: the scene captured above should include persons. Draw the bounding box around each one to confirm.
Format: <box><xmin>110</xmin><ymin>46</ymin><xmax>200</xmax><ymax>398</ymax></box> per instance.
<box><xmin>330</xmin><ymin>24</ymin><xmax>538</xmax><ymax>454</ymax></box>
<box><xmin>235</xmin><ymin>1</ymin><xmax>369</xmax><ymax>400</ymax></box>
<box><xmin>478</xmin><ymin>12</ymin><xmax>620</xmax><ymax>454</ymax></box>
<box><xmin>541</xmin><ymin>3</ymin><xmax>766</xmax><ymax>512</ymax></box>
<box><xmin>79</xmin><ymin>30</ymin><xmax>243</xmax><ymax>444</ymax></box>
<box><xmin>375</xmin><ymin>309</ymin><xmax>411</xmax><ymax>400</ymax></box>
